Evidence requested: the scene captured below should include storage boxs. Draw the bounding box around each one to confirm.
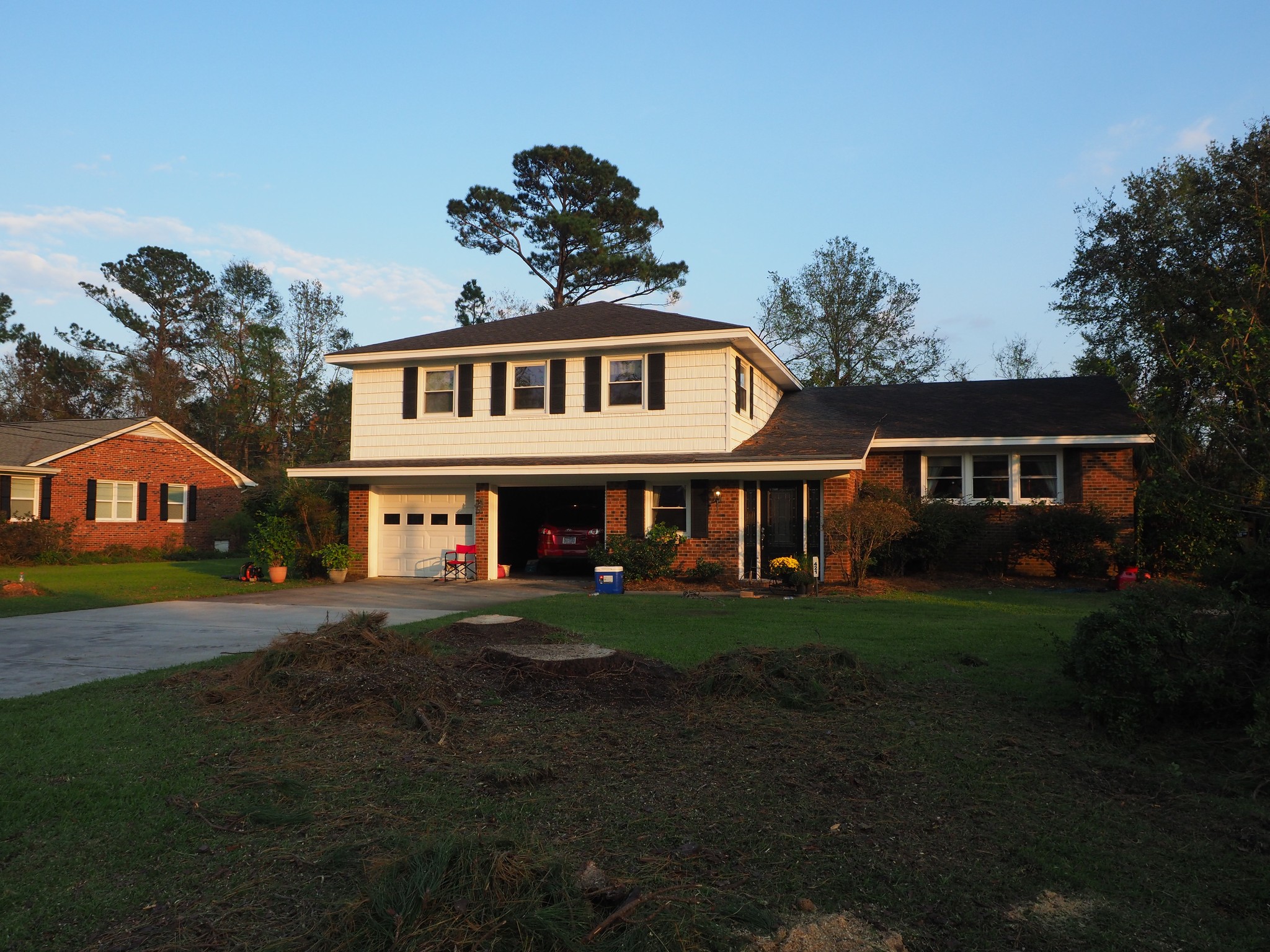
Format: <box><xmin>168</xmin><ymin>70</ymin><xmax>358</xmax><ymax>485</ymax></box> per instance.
<box><xmin>595</xmin><ymin>566</ymin><xmax>623</xmax><ymax>594</ymax></box>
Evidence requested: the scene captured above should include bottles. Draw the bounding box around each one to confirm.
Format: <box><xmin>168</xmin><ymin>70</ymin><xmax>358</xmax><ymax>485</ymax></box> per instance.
<box><xmin>589</xmin><ymin>592</ymin><xmax>599</xmax><ymax>596</ymax></box>
<box><xmin>19</xmin><ymin>572</ymin><xmax>23</xmax><ymax>582</ymax></box>
<box><xmin>783</xmin><ymin>597</ymin><xmax>793</xmax><ymax>600</ymax></box>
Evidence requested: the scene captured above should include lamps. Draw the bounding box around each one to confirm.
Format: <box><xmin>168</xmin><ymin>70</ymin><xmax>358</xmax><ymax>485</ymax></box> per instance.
<box><xmin>712</xmin><ymin>485</ymin><xmax>722</xmax><ymax>510</ymax></box>
<box><xmin>474</xmin><ymin>498</ymin><xmax>483</xmax><ymax>514</ymax></box>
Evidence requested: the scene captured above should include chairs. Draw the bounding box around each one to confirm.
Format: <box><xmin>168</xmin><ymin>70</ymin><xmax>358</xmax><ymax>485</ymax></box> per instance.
<box><xmin>444</xmin><ymin>544</ymin><xmax>476</xmax><ymax>583</ymax></box>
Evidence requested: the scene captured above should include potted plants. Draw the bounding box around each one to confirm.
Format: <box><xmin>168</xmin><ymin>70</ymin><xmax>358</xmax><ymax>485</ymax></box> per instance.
<box><xmin>248</xmin><ymin>516</ymin><xmax>299</xmax><ymax>582</ymax></box>
<box><xmin>311</xmin><ymin>543</ymin><xmax>362</xmax><ymax>583</ymax></box>
<box><xmin>789</xmin><ymin>570</ymin><xmax>815</xmax><ymax>594</ymax></box>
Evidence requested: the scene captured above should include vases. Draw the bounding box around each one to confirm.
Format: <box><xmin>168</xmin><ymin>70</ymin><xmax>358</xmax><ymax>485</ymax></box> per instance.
<box><xmin>782</xmin><ymin>574</ymin><xmax>796</xmax><ymax>587</ymax></box>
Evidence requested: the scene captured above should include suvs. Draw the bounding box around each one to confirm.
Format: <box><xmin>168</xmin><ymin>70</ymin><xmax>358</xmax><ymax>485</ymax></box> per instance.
<box><xmin>537</xmin><ymin>508</ymin><xmax>605</xmax><ymax>567</ymax></box>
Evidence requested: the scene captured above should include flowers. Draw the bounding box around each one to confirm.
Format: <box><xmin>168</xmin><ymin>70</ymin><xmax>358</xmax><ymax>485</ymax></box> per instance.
<box><xmin>768</xmin><ymin>557</ymin><xmax>801</xmax><ymax>576</ymax></box>
